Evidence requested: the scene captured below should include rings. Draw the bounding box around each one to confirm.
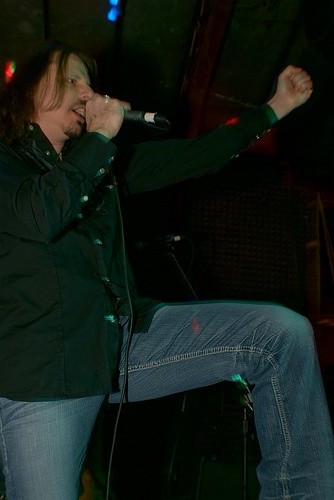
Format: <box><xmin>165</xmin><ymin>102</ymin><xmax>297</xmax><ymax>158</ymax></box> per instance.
<box><xmin>104</xmin><ymin>94</ymin><xmax>109</xmax><ymax>103</ymax></box>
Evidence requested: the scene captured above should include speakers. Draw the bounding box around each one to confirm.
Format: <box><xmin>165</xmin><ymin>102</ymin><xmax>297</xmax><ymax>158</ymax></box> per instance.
<box><xmin>195</xmin><ymin>455</ymin><xmax>261</xmax><ymax>500</ymax></box>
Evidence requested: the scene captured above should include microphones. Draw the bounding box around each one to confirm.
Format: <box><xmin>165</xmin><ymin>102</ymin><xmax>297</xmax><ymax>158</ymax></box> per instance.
<box><xmin>124</xmin><ymin>109</ymin><xmax>166</xmax><ymax>124</ymax></box>
<box><xmin>159</xmin><ymin>234</ymin><xmax>184</xmax><ymax>243</ymax></box>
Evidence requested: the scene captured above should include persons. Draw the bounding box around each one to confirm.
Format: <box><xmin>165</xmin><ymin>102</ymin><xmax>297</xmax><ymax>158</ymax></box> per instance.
<box><xmin>0</xmin><ymin>42</ymin><xmax>334</xmax><ymax>500</ymax></box>
